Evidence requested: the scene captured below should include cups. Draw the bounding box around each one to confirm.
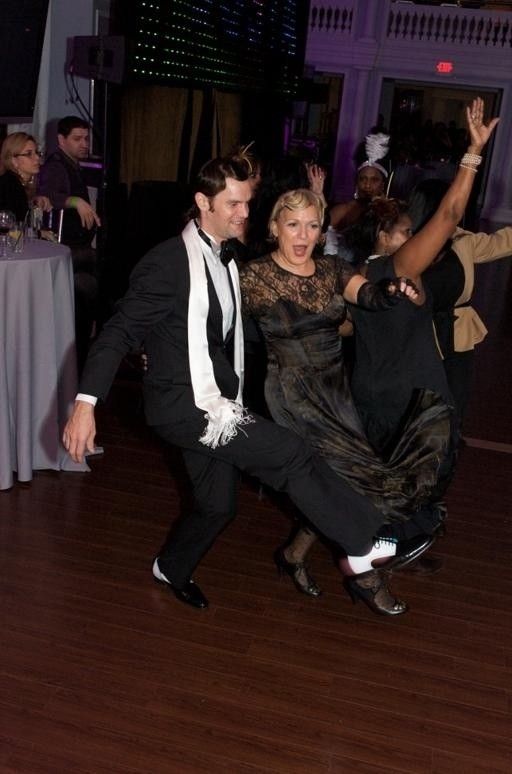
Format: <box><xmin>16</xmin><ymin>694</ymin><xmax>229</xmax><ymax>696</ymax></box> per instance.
<box><xmin>1</xmin><ymin>210</ymin><xmax>12</xmax><ymax>227</ymax></box>
<box><xmin>25</xmin><ymin>226</ymin><xmax>38</xmax><ymax>249</ymax></box>
<box><xmin>0</xmin><ymin>232</ymin><xmax>16</xmax><ymax>260</ymax></box>
<box><xmin>9</xmin><ymin>221</ymin><xmax>24</xmax><ymax>253</ymax></box>
<box><xmin>31</xmin><ymin>207</ymin><xmax>43</xmax><ymax>231</ymax></box>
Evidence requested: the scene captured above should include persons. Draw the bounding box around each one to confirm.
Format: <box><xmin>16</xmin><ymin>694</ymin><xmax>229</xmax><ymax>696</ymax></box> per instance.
<box><xmin>213</xmin><ymin>95</ymin><xmax>512</xmax><ymax>574</ymax></box>
<box><xmin>58</xmin><ymin>140</ymin><xmax>438</xmax><ymax>612</ymax></box>
<box><xmin>36</xmin><ymin>116</ymin><xmax>102</xmax><ymax>377</ymax></box>
<box><xmin>141</xmin><ymin>186</ymin><xmax>423</xmax><ymax>620</ymax></box>
<box><xmin>0</xmin><ymin>130</ymin><xmax>59</xmax><ymax>244</ymax></box>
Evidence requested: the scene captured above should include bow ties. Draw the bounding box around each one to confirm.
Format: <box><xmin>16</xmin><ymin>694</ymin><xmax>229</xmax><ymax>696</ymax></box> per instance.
<box><xmin>193</xmin><ymin>216</ymin><xmax>235</xmax><ymax>266</ymax></box>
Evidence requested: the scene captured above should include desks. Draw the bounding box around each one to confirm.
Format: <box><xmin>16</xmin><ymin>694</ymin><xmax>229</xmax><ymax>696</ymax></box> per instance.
<box><xmin>0</xmin><ymin>236</ymin><xmax>78</xmax><ymax>484</ymax></box>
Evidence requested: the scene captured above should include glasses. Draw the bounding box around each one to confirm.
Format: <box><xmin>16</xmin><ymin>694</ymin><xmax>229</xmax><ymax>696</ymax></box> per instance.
<box><xmin>14</xmin><ymin>152</ymin><xmax>42</xmax><ymax>159</ymax></box>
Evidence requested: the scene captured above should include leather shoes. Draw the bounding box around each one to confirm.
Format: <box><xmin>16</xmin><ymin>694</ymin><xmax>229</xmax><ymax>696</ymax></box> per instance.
<box><xmin>337</xmin><ymin>532</ymin><xmax>437</xmax><ymax>580</ymax></box>
<box><xmin>150</xmin><ymin>555</ymin><xmax>211</xmax><ymax>609</ymax></box>
<box><xmin>385</xmin><ymin>548</ymin><xmax>448</xmax><ymax>578</ymax></box>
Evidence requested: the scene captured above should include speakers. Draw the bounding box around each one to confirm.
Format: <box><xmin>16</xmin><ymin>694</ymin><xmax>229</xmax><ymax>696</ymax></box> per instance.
<box><xmin>72</xmin><ymin>33</ymin><xmax>122</xmax><ymax>83</ymax></box>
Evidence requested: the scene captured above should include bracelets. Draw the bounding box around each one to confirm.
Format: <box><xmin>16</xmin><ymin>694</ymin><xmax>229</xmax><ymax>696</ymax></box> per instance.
<box><xmin>68</xmin><ymin>196</ymin><xmax>78</xmax><ymax>209</ymax></box>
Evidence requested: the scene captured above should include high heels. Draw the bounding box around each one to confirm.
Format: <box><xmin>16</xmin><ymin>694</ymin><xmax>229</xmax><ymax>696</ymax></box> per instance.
<box><xmin>272</xmin><ymin>545</ymin><xmax>326</xmax><ymax>598</ymax></box>
<box><xmin>342</xmin><ymin>570</ymin><xmax>411</xmax><ymax>617</ymax></box>
